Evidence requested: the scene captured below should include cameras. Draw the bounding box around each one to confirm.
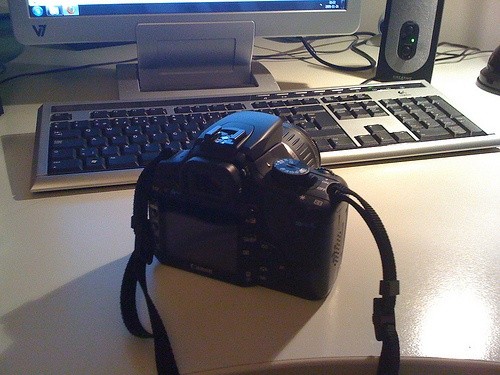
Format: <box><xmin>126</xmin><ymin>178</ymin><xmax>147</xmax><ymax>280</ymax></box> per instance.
<box><xmin>149</xmin><ymin>111</ymin><xmax>349</xmax><ymax>301</ymax></box>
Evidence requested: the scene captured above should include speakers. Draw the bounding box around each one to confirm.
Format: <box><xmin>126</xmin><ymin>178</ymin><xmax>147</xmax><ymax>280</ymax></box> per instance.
<box><xmin>375</xmin><ymin>0</ymin><xmax>446</xmax><ymax>84</ymax></box>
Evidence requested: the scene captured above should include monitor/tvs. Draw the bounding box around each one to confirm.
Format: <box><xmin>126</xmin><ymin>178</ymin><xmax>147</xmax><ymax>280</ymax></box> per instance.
<box><xmin>8</xmin><ymin>0</ymin><xmax>361</xmax><ymax>45</ymax></box>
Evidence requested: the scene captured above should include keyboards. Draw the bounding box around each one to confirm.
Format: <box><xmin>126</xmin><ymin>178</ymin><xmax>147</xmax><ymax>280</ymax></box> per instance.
<box><xmin>30</xmin><ymin>79</ymin><xmax>500</xmax><ymax>194</ymax></box>
